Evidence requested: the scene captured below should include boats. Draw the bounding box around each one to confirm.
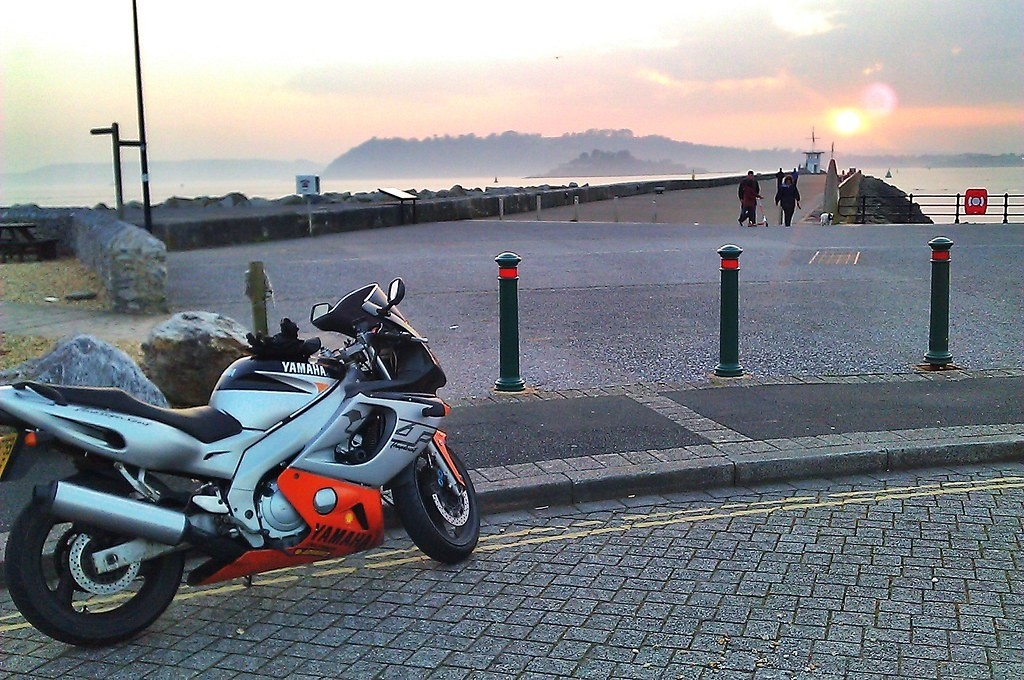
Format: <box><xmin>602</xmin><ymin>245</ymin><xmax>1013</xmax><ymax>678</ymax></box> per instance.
<box><xmin>885</xmin><ymin>169</ymin><xmax>892</xmax><ymax>178</ymax></box>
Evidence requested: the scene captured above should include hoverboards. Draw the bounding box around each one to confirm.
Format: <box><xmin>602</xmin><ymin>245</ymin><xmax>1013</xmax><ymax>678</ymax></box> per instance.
<box><xmin>747</xmin><ymin>196</ymin><xmax>768</xmax><ymax>227</ymax></box>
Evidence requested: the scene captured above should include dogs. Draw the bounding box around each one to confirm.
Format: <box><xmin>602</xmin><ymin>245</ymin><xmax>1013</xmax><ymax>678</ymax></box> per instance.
<box><xmin>820</xmin><ymin>212</ymin><xmax>834</xmax><ymax>226</ymax></box>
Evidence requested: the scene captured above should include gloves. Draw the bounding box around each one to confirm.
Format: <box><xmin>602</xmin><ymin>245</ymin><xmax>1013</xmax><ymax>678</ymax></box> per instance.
<box><xmin>245</xmin><ymin>318</ymin><xmax>321</xmax><ymax>362</ymax></box>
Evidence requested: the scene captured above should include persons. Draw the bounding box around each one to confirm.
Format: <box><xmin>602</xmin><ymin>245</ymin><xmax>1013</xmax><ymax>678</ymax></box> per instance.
<box><xmin>776</xmin><ymin>168</ymin><xmax>784</xmax><ymax>186</ymax></box>
<box><xmin>774</xmin><ymin>175</ymin><xmax>800</xmax><ymax>228</ymax></box>
<box><xmin>792</xmin><ymin>168</ymin><xmax>798</xmax><ymax>186</ymax></box>
<box><xmin>738</xmin><ymin>171</ymin><xmax>764</xmax><ymax>227</ymax></box>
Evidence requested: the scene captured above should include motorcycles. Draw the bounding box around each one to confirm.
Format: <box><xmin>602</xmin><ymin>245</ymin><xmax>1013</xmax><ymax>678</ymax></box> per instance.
<box><xmin>0</xmin><ymin>278</ymin><xmax>481</xmax><ymax>649</ymax></box>
